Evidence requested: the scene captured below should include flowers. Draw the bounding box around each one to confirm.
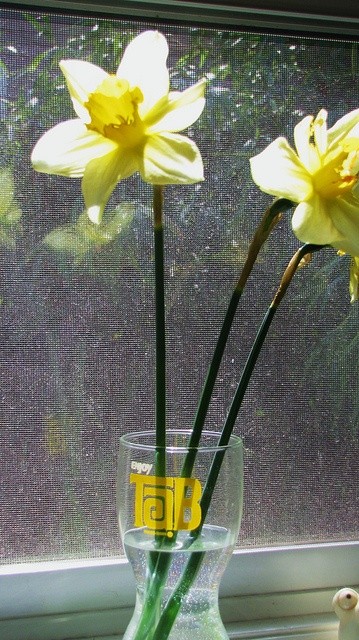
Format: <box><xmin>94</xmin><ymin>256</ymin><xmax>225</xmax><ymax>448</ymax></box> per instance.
<box><xmin>30</xmin><ymin>29</ymin><xmax>358</xmax><ymax>635</ymax></box>
